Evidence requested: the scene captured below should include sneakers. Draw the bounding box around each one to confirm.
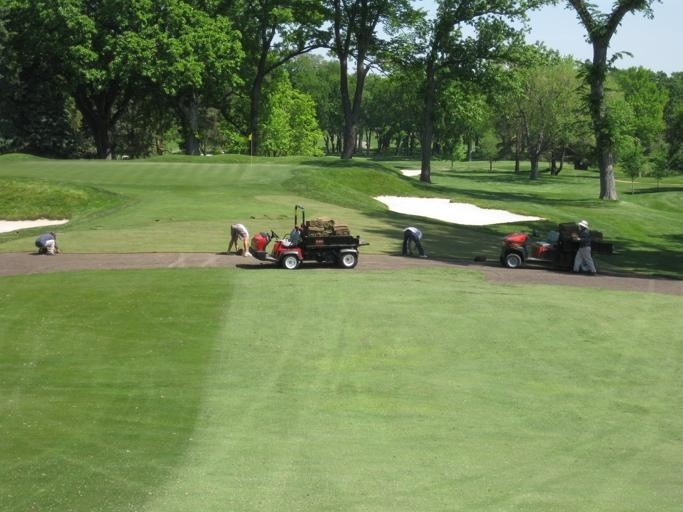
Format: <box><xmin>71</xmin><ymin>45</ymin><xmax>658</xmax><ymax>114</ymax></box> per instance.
<box><xmin>420</xmin><ymin>255</ymin><xmax>428</xmax><ymax>258</ymax></box>
<box><xmin>244</xmin><ymin>252</ymin><xmax>252</xmax><ymax>257</ymax></box>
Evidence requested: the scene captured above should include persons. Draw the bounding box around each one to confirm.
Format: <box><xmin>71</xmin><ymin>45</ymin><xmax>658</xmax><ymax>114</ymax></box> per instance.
<box><xmin>226</xmin><ymin>223</ymin><xmax>252</xmax><ymax>256</ymax></box>
<box><xmin>572</xmin><ymin>219</ymin><xmax>597</xmax><ymax>275</ymax></box>
<box><xmin>402</xmin><ymin>226</ymin><xmax>428</xmax><ymax>257</ymax></box>
<box><xmin>34</xmin><ymin>232</ymin><xmax>60</xmax><ymax>256</ymax></box>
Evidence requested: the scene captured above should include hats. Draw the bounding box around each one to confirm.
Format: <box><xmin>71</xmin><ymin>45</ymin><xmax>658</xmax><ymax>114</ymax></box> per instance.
<box><xmin>579</xmin><ymin>220</ymin><xmax>589</xmax><ymax>229</ymax></box>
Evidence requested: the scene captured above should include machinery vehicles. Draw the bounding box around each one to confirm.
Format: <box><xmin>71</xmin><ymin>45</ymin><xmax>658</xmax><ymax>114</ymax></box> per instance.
<box><xmin>500</xmin><ymin>224</ymin><xmax>614</xmax><ymax>273</ymax></box>
<box><xmin>246</xmin><ymin>203</ymin><xmax>370</xmax><ymax>270</ymax></box>
